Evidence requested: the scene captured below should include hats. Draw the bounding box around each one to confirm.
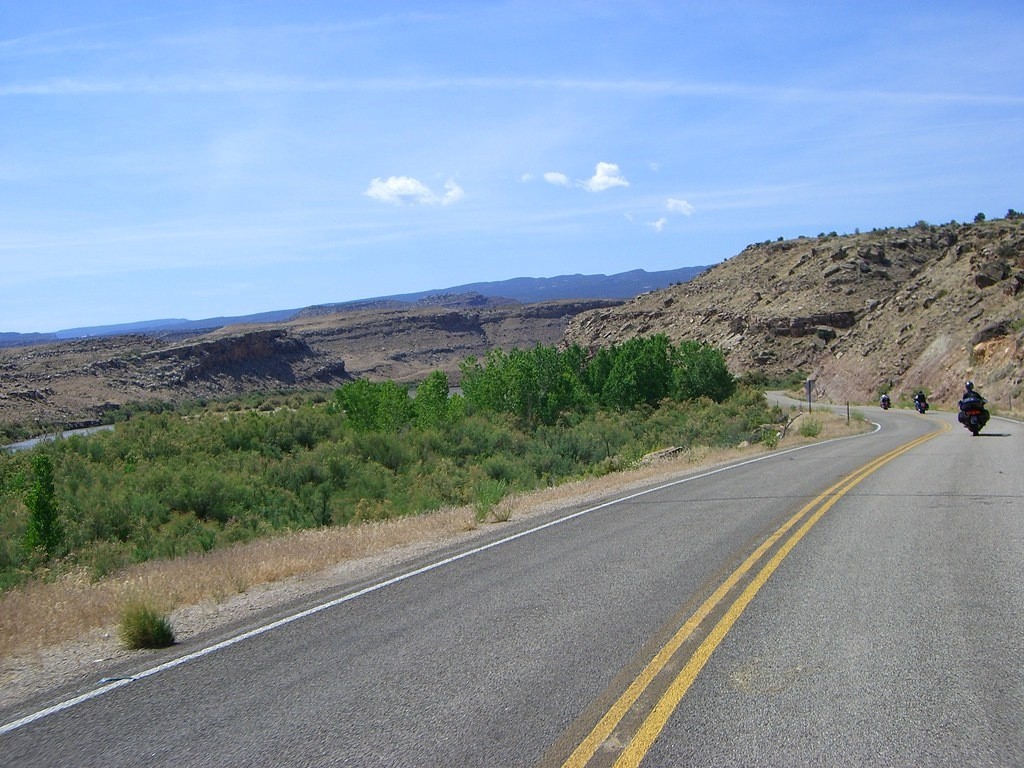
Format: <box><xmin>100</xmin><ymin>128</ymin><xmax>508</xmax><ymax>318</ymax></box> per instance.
<box><xmin>919</xmin><ymin>391</ymin><xmax>922</xmax><ymax>393</ymax></box>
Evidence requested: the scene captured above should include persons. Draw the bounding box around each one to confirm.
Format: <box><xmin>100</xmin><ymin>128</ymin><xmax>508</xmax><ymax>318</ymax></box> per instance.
<box><xmin>881</xmin><ymin>392</ymin><xmax>890</xmax><ymax>402</ymax></box>
<box><xmin>957</xmin><ymin>381</ymin><xmax>989</xmax><ymax>432</ymax></box>
<box><xmin>914</xmin><ymin>391</ymin><xmax>929</xmax><ymax>412</ymax></box>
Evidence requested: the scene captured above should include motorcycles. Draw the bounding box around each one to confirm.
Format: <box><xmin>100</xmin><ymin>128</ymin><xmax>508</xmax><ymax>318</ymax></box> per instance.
<box><xmin>958</xmin><ymin>397</ymin><xmax>990</xmax><ymax>436</ymax></box>
<box><xmin>914</xmin><ymin>398</ymin><xmax>929</xmax><ymax>414</ymax></box>
<box><xmin>881</xmin><ymin>396</ymin><xmax>891</xmax><ymax>410</ymax></box>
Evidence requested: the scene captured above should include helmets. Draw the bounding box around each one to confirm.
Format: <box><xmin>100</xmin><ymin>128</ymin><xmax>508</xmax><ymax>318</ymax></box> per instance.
<box><xmin>884</xmin><ymin>392</ymin><xmax>887</xmax><ymax>394</ymax></box>
<box><xmin>966</xmin><ymin>381</ymin><xmax>974</xmax><ymax>389</ymax></box>
